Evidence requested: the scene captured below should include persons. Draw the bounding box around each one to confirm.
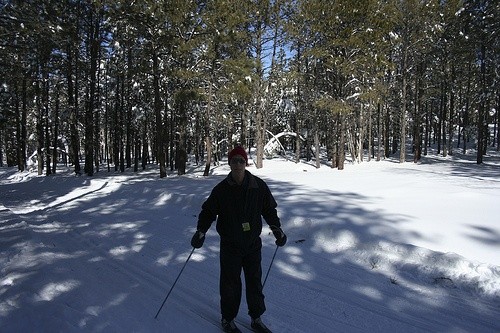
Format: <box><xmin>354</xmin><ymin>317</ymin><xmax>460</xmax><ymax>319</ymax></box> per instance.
<box><xmin>190</xmin><ymin>147</ymin><xmax>287</xmax><ymax>333</ymax></box>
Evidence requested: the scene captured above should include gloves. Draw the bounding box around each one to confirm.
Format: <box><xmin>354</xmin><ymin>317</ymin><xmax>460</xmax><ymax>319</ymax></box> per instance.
<box><xmin>190</xmin><ymin>231</ymin><xmax>205</xmax><ymax>248</ymax></box>
<box><xmin>273</xmin><ymin>228</ymin><xmax>287</xmax><ymax>247</ymax></box>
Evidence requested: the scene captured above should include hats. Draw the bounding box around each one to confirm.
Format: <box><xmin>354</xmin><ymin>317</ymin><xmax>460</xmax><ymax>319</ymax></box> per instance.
<box><xmin>228</xmin><ymin>146</ymin><xmax>248</xmax><ymax>161</ymax></box>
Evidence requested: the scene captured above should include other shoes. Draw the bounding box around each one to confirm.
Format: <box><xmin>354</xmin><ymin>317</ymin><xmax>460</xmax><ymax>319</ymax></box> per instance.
<box><xmin>251</xmin><ymin>318</ymin><xmax>272</xmax><ymax>332</ymax></box>
<box><xmin>221</xmin><ymin>318</ymin><xmax>242</xmax><ymax>333</ymax></box>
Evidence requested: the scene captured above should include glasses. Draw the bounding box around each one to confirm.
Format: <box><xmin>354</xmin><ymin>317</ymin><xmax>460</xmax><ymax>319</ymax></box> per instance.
<box><xmin>231</xmin><ymin>158</ymin><xmax>246</xmax><ymax>164</ymax></box>
